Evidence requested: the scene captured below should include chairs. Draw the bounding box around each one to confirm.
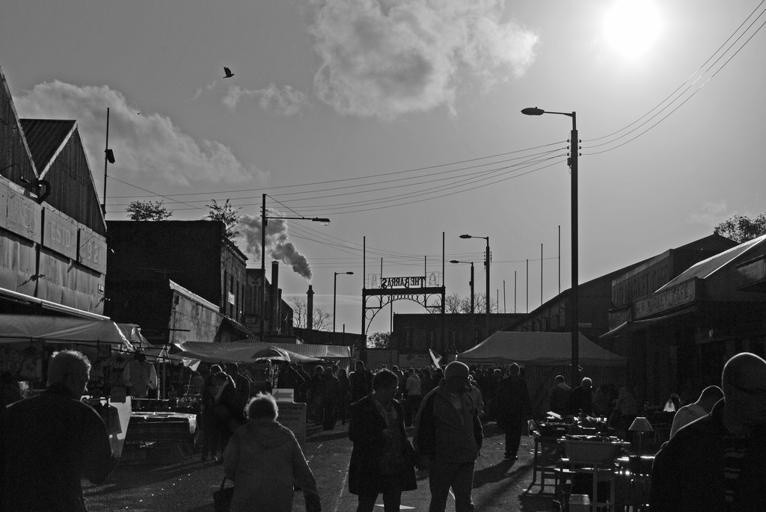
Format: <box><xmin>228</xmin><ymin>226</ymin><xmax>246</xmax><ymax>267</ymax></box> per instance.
<box><xmin>522</xmin><ymin>410</ymin><xmax>659</xmax><ymax>512</ymax></box>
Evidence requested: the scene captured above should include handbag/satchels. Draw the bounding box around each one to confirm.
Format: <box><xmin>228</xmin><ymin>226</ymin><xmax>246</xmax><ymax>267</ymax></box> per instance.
<box><xmin>212</xmin><ymin>476</ymin><xmax>234</xmax><ymax>512</ymax></box>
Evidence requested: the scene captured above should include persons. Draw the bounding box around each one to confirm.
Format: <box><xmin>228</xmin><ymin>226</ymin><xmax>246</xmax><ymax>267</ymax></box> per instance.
<box><xmin>412</xmin><ymin>360</ymin><xmax>484</xmax><ymax>512</ymax></box>
<box><xmin>202</xmin><ymin>362</ymin><xmax>321</xmax><ymax>512</ymax></box>
<box><xmin>548</xmin><ymin>375</ymin><xmax>638</xmax><ymax>442</ymax></box>
<box><xmin>677</xmin><ymin>353</ymin><xmax>765</xmax><ymax>512</ymax></box>
<box><xmin>497</xmin><ymin>363</ymin><xmax>533</xmax><ymax>460</ymax></box>
<box><xmin>1</xmin><ymin>348</ymin><xmax>110</xmax><ymax>512</ymax></box>
<box><xmin>299</xmin><ymin>365</ymin><xmax>507</xmax><ymax>431</ymax></box>
<box><xmin>348</xmin><ymin>367</ymin><xmax>420</xmax><ymax>511</ymax></box>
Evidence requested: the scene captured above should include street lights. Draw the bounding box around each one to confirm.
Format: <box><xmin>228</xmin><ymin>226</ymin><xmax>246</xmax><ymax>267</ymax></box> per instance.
<box><xmin>449</xmin><ymin>259</ymin><xmax>475</xmax><ymax>316</ymax></box>
<box><xmin>258</xmin><ymin>193</ymin><xmax>330</xmax><ymax>340</ymax></box>
<box><xmin>459</xmin><ymin>234</ymin><xmax>493</xmax><ymax>336</ymax></box>
<box><xmin>331</xmin><ymin>271</ymin><xmax>353</xmax><ymax>342</ymax></box>
<box><xmin>520</xmin><ymin>106</ymin><xmax>583</xmax><ymax>391</ymax></box>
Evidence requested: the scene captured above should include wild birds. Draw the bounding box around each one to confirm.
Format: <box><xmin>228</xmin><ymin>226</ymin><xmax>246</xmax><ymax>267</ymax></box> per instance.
<box><xmin>222</xmin><ymin>67</ymin><xmax>235</xmax><ymax>80</ymax></box>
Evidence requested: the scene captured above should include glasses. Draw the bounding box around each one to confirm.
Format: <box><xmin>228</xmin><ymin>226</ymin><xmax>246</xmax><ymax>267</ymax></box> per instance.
<box><xmin>724</xmin><ymin>382</ymin><xmax>765</xmax><ymax>399</ymax></box>
